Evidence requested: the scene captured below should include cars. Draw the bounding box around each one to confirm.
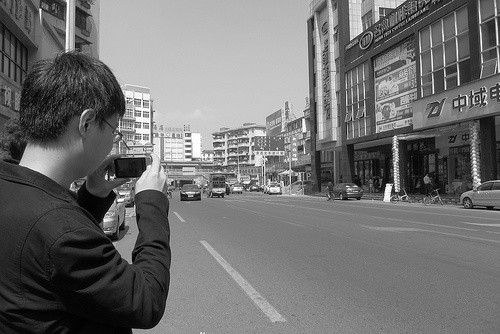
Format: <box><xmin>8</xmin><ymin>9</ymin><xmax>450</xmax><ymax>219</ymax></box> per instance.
<box><xmin>332</xmin><ymin>182</ymin><xmax>364</xmax><ymax>201</ymax></box>
<box><xmin>460</xmin><ymin>180</ymin><xmax>500</xmax><ymax>209</ymax></box>
<box><xmin>98</xmin><ymin>188</ymin><xmax>126</xmax><ymax>239</ymax></box>
<box><xmin>179</xmin><ymin>184</ymin><xmax>201</xmax><ymax>201</ymax></box>
<box><xmin>203</xmin><ymin>173</ymin><xmax>282</xmax><ymax>198</ymax></box>
<box><xmin>117</xmin><ymin>183</ymin><xmax>135</xmax><ymax>207</ymax></box>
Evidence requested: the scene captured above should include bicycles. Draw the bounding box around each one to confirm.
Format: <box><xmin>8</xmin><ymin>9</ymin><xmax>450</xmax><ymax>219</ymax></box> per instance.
<box><xmin>326</xmin><ymin>188</ymin><xmax>336</xmax><ymax>201</ymax></box>
<box><xmin>423</xmin><ymin>188</ymin><xmax>444</xmax><ymax>206</ymax></box>
<box><xmin>389</xmin><ymin>187</ymin><xmax>414</xmax><ymax>203</ymax></box>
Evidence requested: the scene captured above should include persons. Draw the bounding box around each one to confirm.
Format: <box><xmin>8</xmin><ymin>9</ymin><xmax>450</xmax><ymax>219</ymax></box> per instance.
<box><xmin>327</xmin><ymin>180</ymin><xmax>333</xmax><ymax>200</ymax></box>
<box><xmin>423</xmin><ymin>174</ymin><xmax>432</xmax><ymax>196</ymax></box>
<box><xmin>369</xmin><ymin>176</ymin><xmax>379</xmax><ymax>193</ymax></box>
<box><xmin>0</xmin><ymin>52</ymin><xmax>172</xmax><ymax>334</ymax></box>
<box><xmin>0</xmin><ymin>118</ymin><xmax>27</xmax><ymax>164</ymax></box>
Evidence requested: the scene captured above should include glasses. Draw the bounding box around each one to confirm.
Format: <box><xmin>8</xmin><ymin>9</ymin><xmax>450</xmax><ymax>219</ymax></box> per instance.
<box><xmin>95</xmin><ymin>113</ymin><xmax>123</xmax><ymax>143</ymax></box>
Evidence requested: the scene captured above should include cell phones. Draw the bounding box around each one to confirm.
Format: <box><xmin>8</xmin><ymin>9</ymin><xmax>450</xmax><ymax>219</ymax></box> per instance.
<box><xmin>114</xmin><ymin>157</ymin><xmax>146</xmax><ymax>178</ymax></box>
